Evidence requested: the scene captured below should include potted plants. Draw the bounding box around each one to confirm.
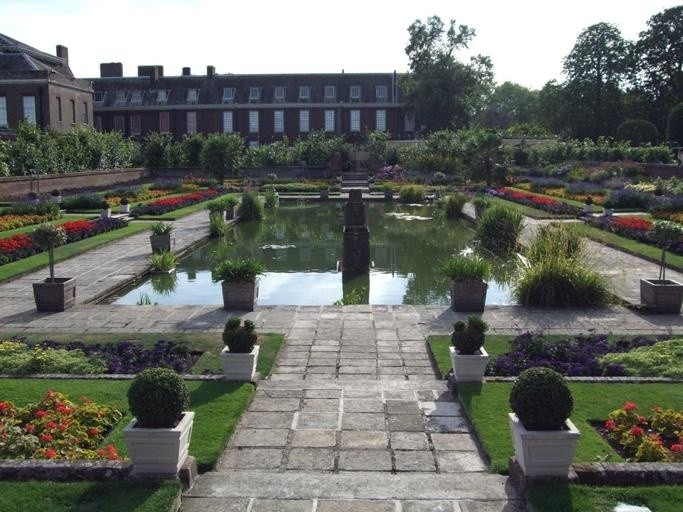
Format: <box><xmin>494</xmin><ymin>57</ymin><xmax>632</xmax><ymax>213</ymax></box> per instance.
<box><xmin>26</xmin><ymin>190</ymin><xmax>130</xmax><ymax>218</ymax></box>
<box><xmin>450</xmin><ymin>314</ymin><xmax>491</xmax><ymax>383</ymax></box>
<box><xmin>472</xmin><ymin>196</ymin><xmax>492</xmax><ymax>220</ymax></box>
<box><xmin>120</xmin><ymin>367</ymin><xmax>196</xmax><ymax>479</ymax></box>
<box><xmin>320</xmin><ymin>172</ymin><xmax>393</xmax><ymax>200</ymax></box>
<box><xmin>507</xmin><ymin>365</ymin><xmax>582</xmax><ymax>479</ymax></box>
<box><xmin>583</xmin><ymin>194</ymin><xmax>615</xmax><ymax>216</ymax></box>
<box><xmin>32</xmin><ymin>221</ymin><xmax>77</xmax><ymax>311</ymax></box>
<box><xmin>206</xmin><ymin>195</ymin><xmax>241</xmax><ymax>223</ymax></box>
<box><xmin>440</xmin><ymin>252</ymin><xmax>495</xmax><ymax>312</ymax></box>
<box><xmin>220</xmin><ymin>317</ymin><xmax>260</xmax><ymax>382</ymax></box>
<box><xmin>640</xmin><ymin>219</ymin><xmax>683</xmax><ymax>314</ymax></box>
<box><xmin>212</xmin><ymin>255</ymin><xmax>267</xmax><ymax>312</ymax></box>
